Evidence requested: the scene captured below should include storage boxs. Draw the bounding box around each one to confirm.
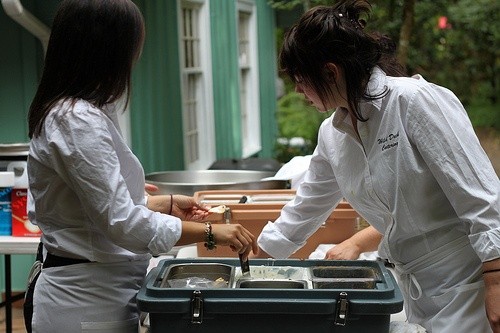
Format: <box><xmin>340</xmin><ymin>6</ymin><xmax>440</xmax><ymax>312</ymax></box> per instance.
<box><xmin>194</xmin><ymin>190</ymin><xmax>371</xmax><ymax>258</ymax></box>
<box><xmin>136</xmin><ymin>257</ymin><xmax>405</xmax><ymax>333</ymax></box>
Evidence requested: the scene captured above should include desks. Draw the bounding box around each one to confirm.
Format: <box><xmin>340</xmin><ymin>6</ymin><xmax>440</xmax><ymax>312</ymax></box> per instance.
<box><xmin>0</xmin><ymin>236</ymin><xmax>41</xmax><ymax>332</ymax></box>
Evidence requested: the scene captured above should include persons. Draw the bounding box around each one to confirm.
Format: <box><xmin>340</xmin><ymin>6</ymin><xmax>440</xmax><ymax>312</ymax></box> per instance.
<box><xmin>324</xmin><ymin>225</ymin><xmax>383</xmax><ymax>260</ymax></box>
<box><xmin>248</xmin><ymin>0</ymin><xmax>500</xmax><ymax>333</ymax></box>
<box><xmin>23</xmin><ymin>0</ymin><xmax>260</xmax><ymax>333</ymax></box>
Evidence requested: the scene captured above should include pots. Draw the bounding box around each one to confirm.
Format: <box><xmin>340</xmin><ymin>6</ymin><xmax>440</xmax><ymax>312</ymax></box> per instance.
<box><xmin>145</xmin><ymin>170</ymin><xmax>290</xmax><ymax>196</ymax></box>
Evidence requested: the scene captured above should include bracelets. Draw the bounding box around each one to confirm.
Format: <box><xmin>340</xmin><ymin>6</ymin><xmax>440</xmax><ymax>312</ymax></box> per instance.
<box><xmin>169</xmin><ymin>193</ymin><xmax>174</xmax><ymax>215</ymax></box>
<box><xmin>482</xmin><ymin>268</ymin><xmax>500</xmax><ymax>274</ymax></box>
<box><xmin>204</xmin><ymin>221</ymin><xmax>217</xmax><ymax>250</ymax></box>
